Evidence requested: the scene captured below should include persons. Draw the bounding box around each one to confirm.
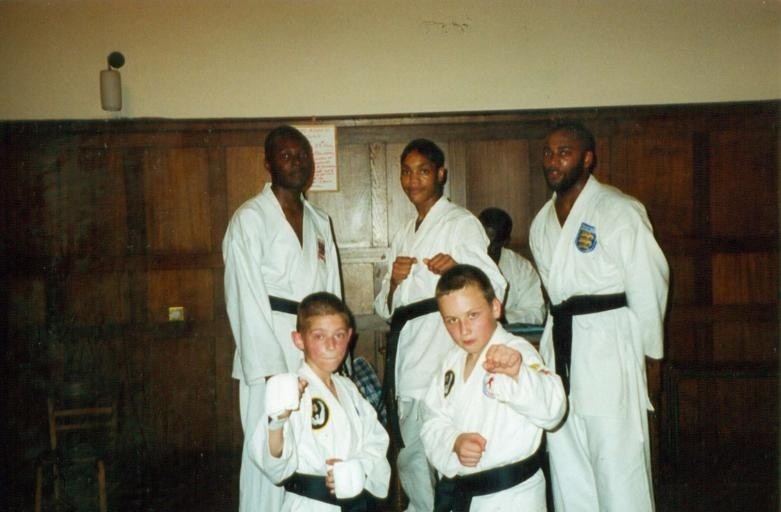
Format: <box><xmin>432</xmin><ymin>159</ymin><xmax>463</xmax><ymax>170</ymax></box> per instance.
<box><xmin>261</xmin><ymin>293</ymin><xmax>389</xmax><ymax>512</ymax></box>
<box><xmin>395</xmin><ymin>265</ymin><xmax>566</xmax><ymax>512</ymax></box>
<box><xmin>528</xmin><ymin>124</ymin><xmax>669</xmax><ymax>512</ymax></box>
<box><xmin>372</xmin><ymin>140</ymin><xmax>505</xmax><ymax>442</ymax></box>
<box><xmin>476</xmin><ymin>207</ymin><xmax>545</xmax><ymax>344</ymax></box>
<box><xmin>220</xmin><ymin>126</ymin><xmax>342</xmax><ymax>512</ymax></box>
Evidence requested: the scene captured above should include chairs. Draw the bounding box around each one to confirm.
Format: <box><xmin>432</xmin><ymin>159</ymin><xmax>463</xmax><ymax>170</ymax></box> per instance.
<box><xmin>32</xmin><ymin>383</ymin><xmax>123</xmax><ymax>511</ymax></box>
